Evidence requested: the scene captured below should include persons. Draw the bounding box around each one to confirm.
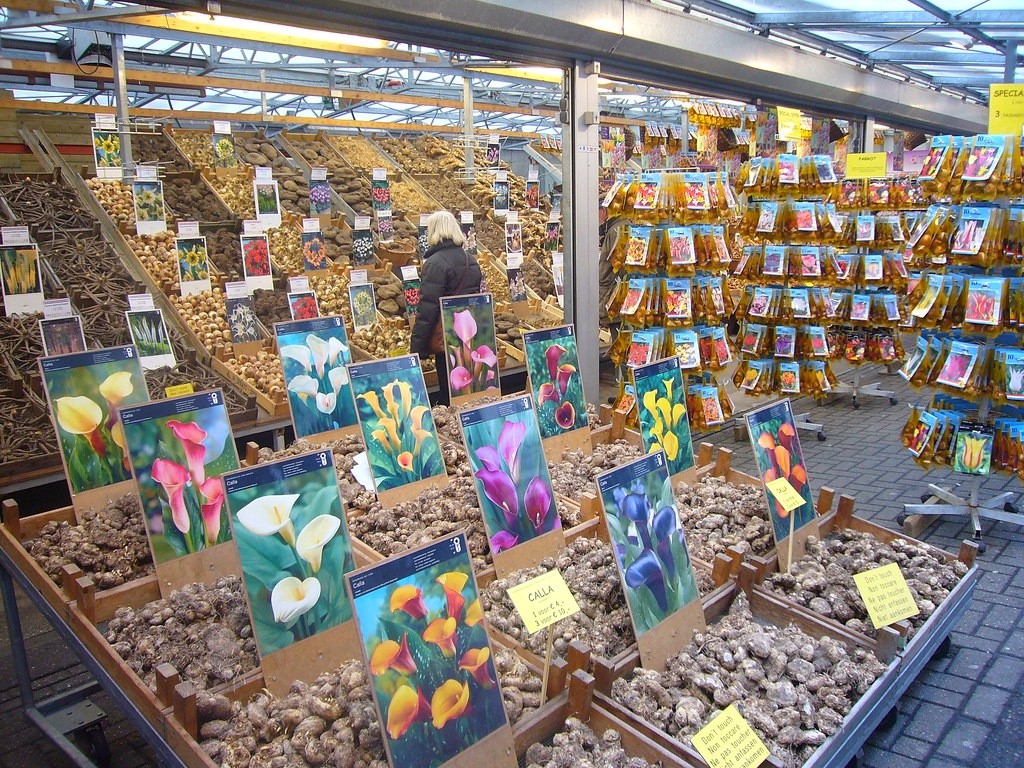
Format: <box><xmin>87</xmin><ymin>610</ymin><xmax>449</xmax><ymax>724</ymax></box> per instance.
<box><xmin>410</xmin><ymin>209</ymin><xmax>482</xmax><ymax>407</ymax></box>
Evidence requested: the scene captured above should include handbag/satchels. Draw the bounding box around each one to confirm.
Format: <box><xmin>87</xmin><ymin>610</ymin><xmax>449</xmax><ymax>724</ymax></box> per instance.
<box><xmin>426</xmin><ymin>314</ymin><xmax>445</xmax><ymax>353</ymax></box>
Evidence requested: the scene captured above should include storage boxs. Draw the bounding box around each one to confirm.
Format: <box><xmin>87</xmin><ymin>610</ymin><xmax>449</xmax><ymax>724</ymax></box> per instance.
<box><xmin>1</xmin><ymin>291</ymin><xmax>101</xmax><ymax>391</ymax></box>
<box><xmin>749</xmin><ymin>493</ymin><xmax>981</xmax><ymax>675</ymax></box>
<box><xmin>477</xmin><ymin>489</ymin><xmax>737</xmax><ymax>687</ymax></box>
<box><xmin>553</xmin><ymin>408</ymin><xmax>714</xmax><ymax>517</ymax></box>
<box><xmin>348</xmin><ymin>469</ymin><xmax>601</xmax><ymax>589</ymax></box>
<box><xmin>242</xmin><ymin>423</ymin><xmax>467</xmax><ymax>509</ymax></box>
<box><xmin>30</xmin><ymin>221</ymin><xmax>144</xmax><ymax>298</ymax></box>
<box><xmin>508</xmin><ymin>666</ymin><xmax>697</xmax><ymax>767</ymax></box>
<box><xmin>74</xmin><ymin>284</ymin><xmax>186</xmax><ymax>362</ymax></box>
<box><xmin>77</xmin><ymin>132</ymin><xmax>565</xmax><ymax>417</ymax></box>
<box><xmin>431</xmin><ymin>374</ymin><xmax>614</xmax><ymax>451</ymax></box>
<box><xmin>589</xmin><ymin>562</ymin><xmax>905</xmax><ymax>768</ymax></box>
<box><xmin>0</xmin><ymin>463</ymin><xmax>245</xmax><ymax>627</ymax></box>
<box><xmin>1</xmin><ymin>164</ymin><xmax>100</xmax><ymax>244</ymax></box>
<box><xmin>1</xmin><ymin>237</ymin><xmax>64</xmax><ymax>316</ymax></box>
<box><xmin>1</xmin><ymin>377</ymin><xmax>63</xmax><ymax>479</ymax></box>
<box><xmin>165</xmin><ymin>634</ymin><xmax>571</xmax><ymax>768</ymax></box>
<box><xmin>66</xmin><ymin>498</ymin><xmax>374</xmax><ymax>734</ymax></box>
<box><xmin>693</xmin><ymin>446</ymin><xmax>837</xmax><ymax>580</ymax></box>
